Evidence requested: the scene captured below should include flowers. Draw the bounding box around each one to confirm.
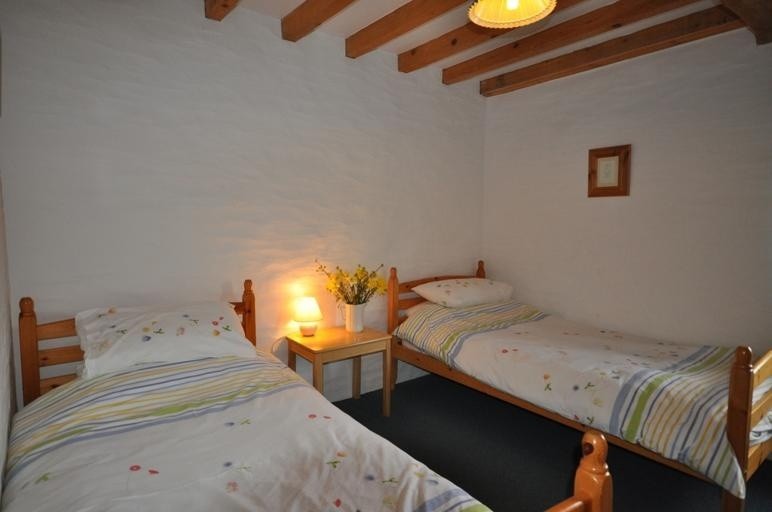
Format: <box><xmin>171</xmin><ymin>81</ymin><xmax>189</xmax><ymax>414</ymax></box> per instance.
<box><xmin>314</xmin><ymin>257</ymin><xmax>392</xmax><ymax>307</ymax></box>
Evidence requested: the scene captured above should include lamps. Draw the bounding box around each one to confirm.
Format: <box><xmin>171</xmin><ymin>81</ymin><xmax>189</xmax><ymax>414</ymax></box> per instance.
<box><xmin>468</xmin><ymin>0</ymin><xmax>559</xmax><ymax>30</ymax></box>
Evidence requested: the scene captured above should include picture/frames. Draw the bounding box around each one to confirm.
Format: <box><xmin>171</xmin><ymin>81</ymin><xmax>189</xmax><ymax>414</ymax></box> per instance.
<box><xmin>586</xmin><ymin>142</ymin><xmax>632</xmax><ymax>198</ymax></box>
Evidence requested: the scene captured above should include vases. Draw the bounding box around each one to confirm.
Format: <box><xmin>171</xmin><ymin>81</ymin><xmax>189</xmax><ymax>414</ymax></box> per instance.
<box><xmin>344</xmin><ymin>301</ymin><xmax>366</xmax><ymax>333</ymax></box>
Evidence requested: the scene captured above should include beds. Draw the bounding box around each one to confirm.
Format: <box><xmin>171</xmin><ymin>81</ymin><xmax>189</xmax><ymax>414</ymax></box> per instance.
<box><xmin>2</xmin><ymin>276</ymin><xmax>616</xmax><ymax>511</ymax></box>
<box><xmin>389</xmin><ymin>255</ymin><xmax>771</xmax><ymax>512</ymax></box>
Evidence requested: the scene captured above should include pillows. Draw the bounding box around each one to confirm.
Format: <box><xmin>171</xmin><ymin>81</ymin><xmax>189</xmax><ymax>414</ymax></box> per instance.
<box><xmin>73</xmin><ymin>301</ymin><xmax>257</xmax><ymax>381</ymax></box>
<box><xmin>409</xmin><ymin>277</ymin><xmax>516</xmax><ymax>312</ymax></box>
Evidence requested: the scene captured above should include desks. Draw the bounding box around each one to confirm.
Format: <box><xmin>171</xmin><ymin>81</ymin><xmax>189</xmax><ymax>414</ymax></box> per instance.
<box><xmin>282</xmin><ymin>322</ymin><xmax>393</xmax><ymax>419</ymax></box>
